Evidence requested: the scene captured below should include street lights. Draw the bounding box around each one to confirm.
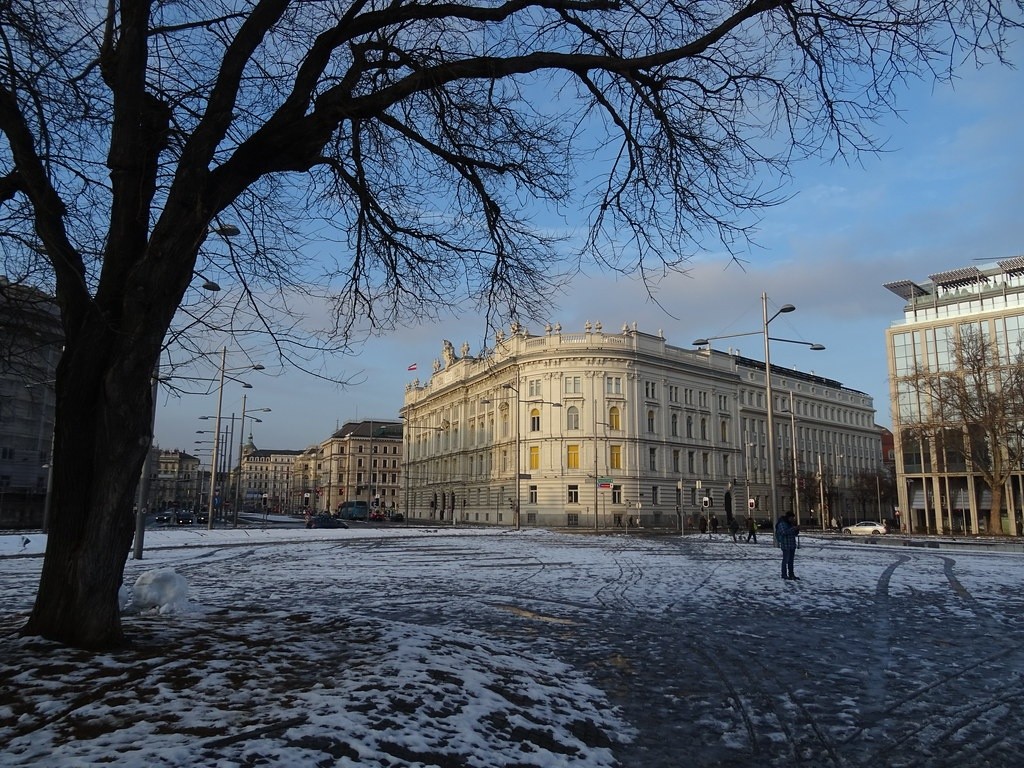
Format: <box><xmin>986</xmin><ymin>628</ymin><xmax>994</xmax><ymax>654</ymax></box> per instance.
<box><xmin>192</xmin><ymin>393</ymin><xmax>272</xmax><ymax>528</ymax></box>
<box><xmin>158</xmin><ymin>346</ymin><xmax>265</xmax><ymax>531</ymax></box>
<box><xmin>480</xmin><ymin>366</ymin><xmax>562</xmax><ymax>532</ymax></box>
<box><xmin>592</xmin><ymin>398</ymin><xmax>614</xmax><ymax>529</ymax></box>
<box><xmin>780</xmin><ymin>390</ymin><xmax>801</xmax><ymax>526</ymax></box>
<box><xmin>398</xmin><ymin>404</ymin><xmax>409</xmax><ymax>525</ymax></box>
<box><xmin>24</xmin><ymin>379</ymin><xmax>56</xmax><ymax>534</ymax></box>
<box><xmin>695</xmin><ymin>293</ymin><xmax>827</xmax><ymax>548</ymax></box>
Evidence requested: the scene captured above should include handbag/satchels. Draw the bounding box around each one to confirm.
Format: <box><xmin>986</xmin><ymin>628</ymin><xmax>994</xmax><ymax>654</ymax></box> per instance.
<box><xmin>753</xmin><ymin>521</ymin><xmax>757</xmax><ymax>531</ymax></box>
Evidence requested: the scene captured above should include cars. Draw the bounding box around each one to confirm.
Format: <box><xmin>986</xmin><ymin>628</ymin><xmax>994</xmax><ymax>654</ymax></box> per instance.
<box><xmin>307</xmin><ymin>516</ymin><xmax>347</xmax><ymax>528</ymax></box>
<box><xmin>841</xmin><ymin>520</ymin><xmax>889</xmax><ymax>535</ymax></box>
<box><xmin>156</xmin><ymin>505</ymin><xmax>215</xmax><ymax>525</ymax></box>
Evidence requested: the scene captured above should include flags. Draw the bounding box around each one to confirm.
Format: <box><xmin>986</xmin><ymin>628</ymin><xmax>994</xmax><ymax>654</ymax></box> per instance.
<box><xmin>408</xmin><ymin>364</ymin><xmax>416</xmax><ymax>370</ymax></box>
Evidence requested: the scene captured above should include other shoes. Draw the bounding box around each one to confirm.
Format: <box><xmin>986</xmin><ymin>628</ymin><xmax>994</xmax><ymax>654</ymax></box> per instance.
<box><xmin>788</xmin><ymin>575</ymin><xmax>800</xmax><ymax>580</ymax></box>
<box><xmin>782</xmin><ymin>573</ymin><xmax>789</xmax><ymax>579</ymax></box>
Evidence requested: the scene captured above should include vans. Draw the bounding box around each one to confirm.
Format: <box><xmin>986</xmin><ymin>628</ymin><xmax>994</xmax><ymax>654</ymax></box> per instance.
<box><xmin>337</xmin><ymin>499</ymin><xmax>369</xmax><ymax>519</ymax></box>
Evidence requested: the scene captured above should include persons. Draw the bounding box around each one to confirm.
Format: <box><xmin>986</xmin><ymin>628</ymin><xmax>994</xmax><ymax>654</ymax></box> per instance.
<box><xmin>710</xmin><ymin>515</ymin><xmax>718</xmax><ymax>534</ymax></box>
<box><xmin>730</xmin><ymin>517</ymin><xmax>739</xmax><ymax>543</ymax></box>
<box><xmin>628</xmin><ymin>515</ymin><xmax>635</xmax><ymax>527</ymax></box>
<box><xmin>775</xmin><ymin>510</ymin><xmax>800</xmax><ymax>580</ymax></box>
<box><xmin>617</xmin><ymin>515</ymin><xmax>622</xmax><ymax>527</ymax></box>
<box><xmin>688</xmin><ymin>515</ymin><xmax>693</xmax><ymax>531</ymax></box>
<box><xmin>699</xmin><ymin>516</ymin><xmax>708</xmax><ymax>534</ymax></box>
<box><xmin>746</xmin><ymin>520</ymin><xmax>761</xmax><ymax>544</ymax></box>
